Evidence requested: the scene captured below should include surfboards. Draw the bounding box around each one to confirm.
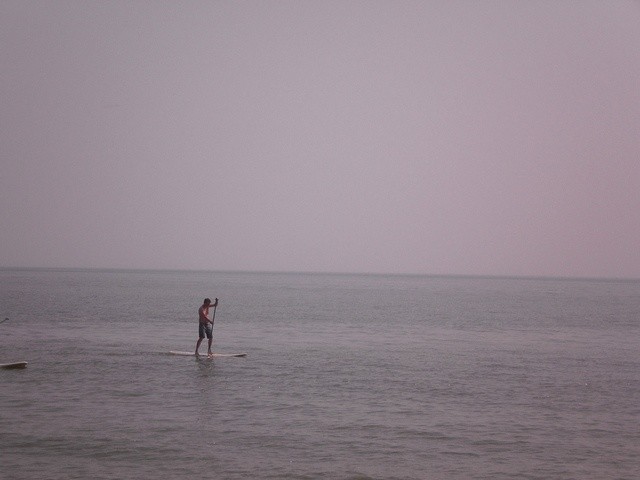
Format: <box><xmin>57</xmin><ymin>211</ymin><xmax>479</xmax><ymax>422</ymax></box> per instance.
<box><xmin>0</xmin><ymin>361</ymin><xmax>28</xmax><ymax>368</ymax></box>
<box><xmin>168</xmin><ymin>350</ymin><xmax>248</xmax><ymax>357</ymax></box>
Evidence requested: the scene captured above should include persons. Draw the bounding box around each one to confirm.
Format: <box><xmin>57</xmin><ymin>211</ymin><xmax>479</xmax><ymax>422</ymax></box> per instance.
<box><xmin>194</xmin><ymin>298</ymin><xmax>218</xmax><ymax>356</ymax></box>
